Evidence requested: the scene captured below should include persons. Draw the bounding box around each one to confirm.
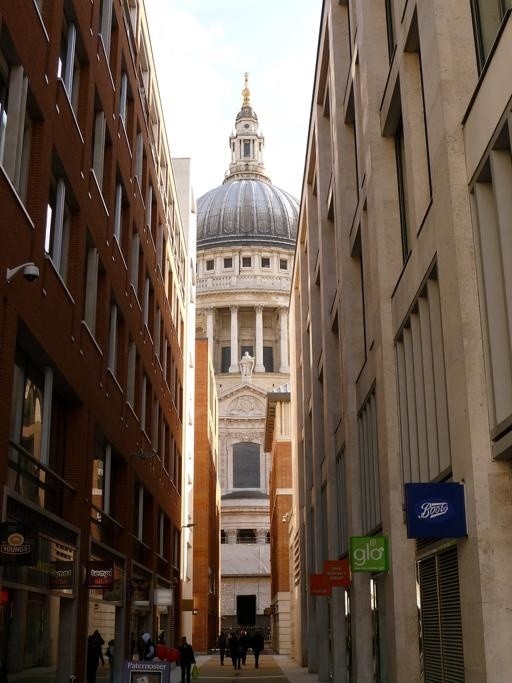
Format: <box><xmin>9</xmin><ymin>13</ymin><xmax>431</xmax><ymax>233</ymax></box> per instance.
<box><xmin>86</xmin><ymin>628</ymin><xmax>265</xmax><ymax>682</ymax></box>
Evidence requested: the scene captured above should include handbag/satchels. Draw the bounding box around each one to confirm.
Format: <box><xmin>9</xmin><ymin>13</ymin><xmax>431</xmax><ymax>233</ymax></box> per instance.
<box><xmin>192</xmin><ymin>664</ymin><xmax>199</xmax><ymax>680</ymax></box>
